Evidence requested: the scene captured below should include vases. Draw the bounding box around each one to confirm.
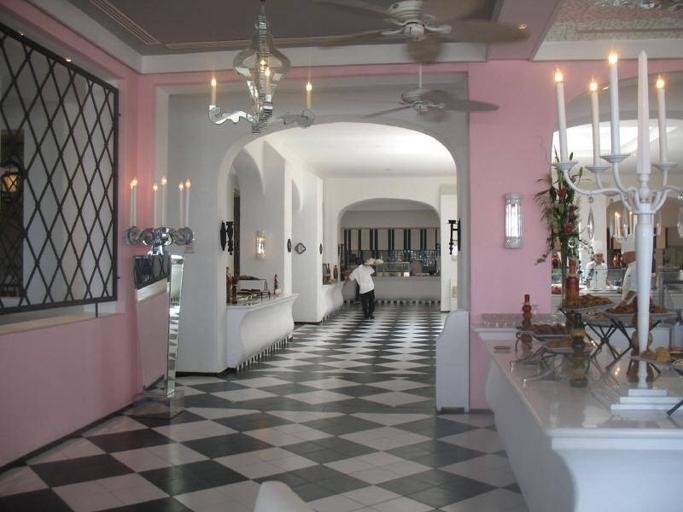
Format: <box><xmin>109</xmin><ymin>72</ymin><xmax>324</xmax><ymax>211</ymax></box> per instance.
<box><xmin>559</xmin><ymin>231</ymin><xmax>579</xmax><ymax>307</ymax></box>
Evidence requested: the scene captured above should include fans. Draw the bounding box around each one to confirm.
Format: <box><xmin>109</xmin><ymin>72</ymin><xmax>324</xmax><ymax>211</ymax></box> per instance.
<box><xmin>361</xmin><ymin>63</ymin><xmax>500</xmax><ymax>122</ymax></box>
<box><xmin>308</xmin><ymin>1</ymin><xmax>528</xmax><ymax>64</ymax></box>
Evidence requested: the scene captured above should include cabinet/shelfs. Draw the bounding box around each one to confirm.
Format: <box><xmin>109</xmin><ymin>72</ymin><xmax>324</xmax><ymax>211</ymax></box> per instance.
<box><xmin>347</xmin><ymin>228</ymin><xmax>439</xmax><ymax>251</ymax></box>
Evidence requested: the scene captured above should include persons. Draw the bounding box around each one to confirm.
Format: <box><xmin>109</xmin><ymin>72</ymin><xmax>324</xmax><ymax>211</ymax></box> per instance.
<box><xmin>344</xmin><ymin>259</ymin><xmax>375</xmax><ymax>319</ymax></box>
<box><xmin>621</xmin><ymin>252</ymin><xmax>636</xmax><ymax>304</ymax></box>
<box><xmin>587</xmin><ymin>254</ymin><xmax>611</xmax><ymax>290</ymax></box>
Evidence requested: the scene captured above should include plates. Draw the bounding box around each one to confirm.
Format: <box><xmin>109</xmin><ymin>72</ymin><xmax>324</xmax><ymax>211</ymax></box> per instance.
<box><xmin>604</xmin><ymin>310</ymin><xmax>677</xmax><ymax>323</ymax></box>
<box><xmin>557</xmin><ymin>302</ymin><xmax>615</xmax><ymax>315</ymax></box>
<box><xmin>512</xmin><ymin>325</ymin><xmax>594</xmax><ymax>355</ymax></box>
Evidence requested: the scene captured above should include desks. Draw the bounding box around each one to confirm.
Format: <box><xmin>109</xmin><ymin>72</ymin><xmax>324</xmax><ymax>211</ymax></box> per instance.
<box><xmin>226</xmin><ymin>293</ymin><xmax>299</xmax><ymax>370</ymax></box>
<box><xmin>470</xmin><ymin>321</ymin><xmax>673</xmax><ymax>414</ymax></box>
<box><xmin>481</xmin><ymin>340</ymin><xmax>683</xmax><ymax>512</ymax></box>
<box><xmin>321</xmin><ymin>280</ymin><xmax>346</xmax><ymax>321</ymax></box>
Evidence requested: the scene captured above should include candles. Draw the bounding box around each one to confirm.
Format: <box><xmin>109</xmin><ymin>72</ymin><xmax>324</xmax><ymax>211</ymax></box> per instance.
<box><xmin>130</xmin><ymin>177</ymin><xmax>191</xmax><ymax>228</ymax></box>
<box><xmin>552</xmin><ymin>48</ymin><xmax>683</xmax><ymax>172</ymax></box>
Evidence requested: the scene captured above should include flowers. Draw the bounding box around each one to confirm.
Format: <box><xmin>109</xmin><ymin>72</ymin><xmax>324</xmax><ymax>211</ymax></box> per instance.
<box><xmin>534</xmin><ymin>146</ymin><xmax>595</xmax><ymax>270</ymax></box>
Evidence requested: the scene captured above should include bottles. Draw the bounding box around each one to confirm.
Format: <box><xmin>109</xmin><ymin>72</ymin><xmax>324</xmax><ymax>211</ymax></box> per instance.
<box><xmin>334</xmin><ymin>265</ymin><xmax>338</xmax><ymax>280</ymax></box>
<box><xmin>225</xmin><ymin>267</ymin><xmax>230</xmax><ymax>302</ymax></box>
<box><xmin>327</xmin><ymin>263</ymin><xmax>330</xmax><ymax>280</ymax></box>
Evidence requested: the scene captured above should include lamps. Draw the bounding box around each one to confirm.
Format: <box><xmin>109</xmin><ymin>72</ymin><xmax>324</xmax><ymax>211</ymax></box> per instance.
<box><xmin>503</xmin><ymin>192</ymin><xmax>523</xmax><ymax>248</ymax></box>
<box><xmin>207</xmin><ymin>1</ymin><xmax>314</xmax><ymax>135</ymax></box>
